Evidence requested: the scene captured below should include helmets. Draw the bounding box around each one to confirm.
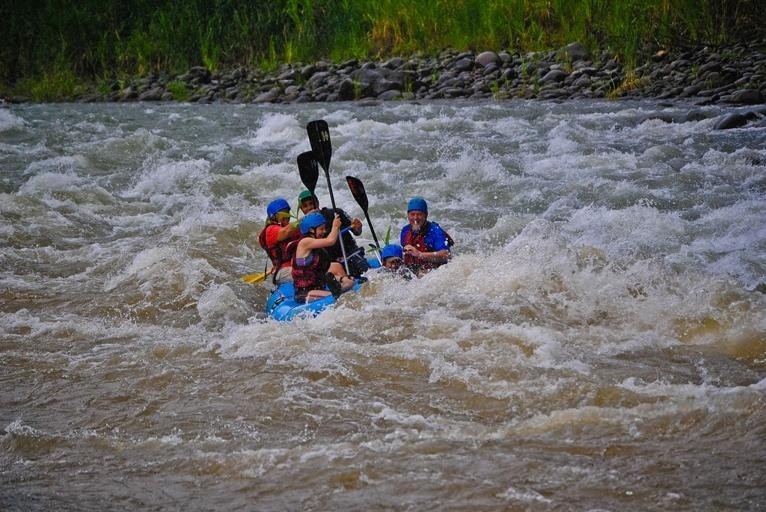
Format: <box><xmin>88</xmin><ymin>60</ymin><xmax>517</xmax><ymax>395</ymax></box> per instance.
<box><xmin>299</xmin><ymin>212</ymin><xmax>327</xmax><ymax>234</ymax></box>
<box><xmin>408</xmin><ymin>197</ymin><xmax>427</xmax><ymax>214</ymax></box>
<box><xmin>382</xmin><ymin>244</ymin><xmax>402</xmax><ymax>258</ymax></box>
<box><xmin>267</xmin><ymin>199</ymin><xmax>291</xmax><ymax>221</ymax></box>
<box><xmin>298</xmin><ymin>192</ymin><xmax>318</xmax><ymax>209</ymax></box>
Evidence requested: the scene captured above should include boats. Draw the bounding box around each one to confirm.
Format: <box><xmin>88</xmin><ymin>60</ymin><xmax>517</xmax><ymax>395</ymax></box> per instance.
<box><xmin>266</xmin><ymin>271</ymin><xmax>417</xmax><ymax>325</ymax></box>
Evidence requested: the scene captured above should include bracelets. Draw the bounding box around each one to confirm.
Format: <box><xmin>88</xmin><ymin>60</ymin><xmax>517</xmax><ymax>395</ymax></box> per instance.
<box><xmin>339</xmin><ymin>276</ymin><xmax>344</xmax><ymax>283</ymax></box>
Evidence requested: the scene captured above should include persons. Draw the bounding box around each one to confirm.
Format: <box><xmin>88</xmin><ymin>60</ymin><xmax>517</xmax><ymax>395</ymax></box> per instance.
<box><xmin>256</xmin><ymin>197</ymin><xmax>350</xmax><ymax>284</ymax></box>
<box><xmin>296</xmin><ymin>189</ymin><xmax>364</xmax><ymax>276</ymax></box>
<box><xmin>375</xmin><ymin>243</ymin><xmax>429</xmax><ymax>282</ymax></box>
<box><xmin>400</xmin><ymin>198</ymin><xmax>455</xmax><ymax>278</ymax></box>
<box><xmin>288</xmin><ymin>210</ymin><xmax>357</xmax><ymax>306</ymax></box>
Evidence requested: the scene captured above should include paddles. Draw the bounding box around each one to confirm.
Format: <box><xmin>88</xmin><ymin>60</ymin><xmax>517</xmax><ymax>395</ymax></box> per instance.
<box><xmin>241</xmin><ymin>270</ymin><xmax>274</xmax><ymax>283</ymax></box>
<box><xmin>306</xmin><ymin>119</ymin><xmax>351</xmax><ymax>275</ymax></box>
<box><xmin>297</xmin><ymin>151</ymin><xmax>318</xmax><ymax>210</ymax></box>
<box><xmin>346</xmin><ymin>176</ymin><xmax>382</xmax><ymax>259</ymax></box>
